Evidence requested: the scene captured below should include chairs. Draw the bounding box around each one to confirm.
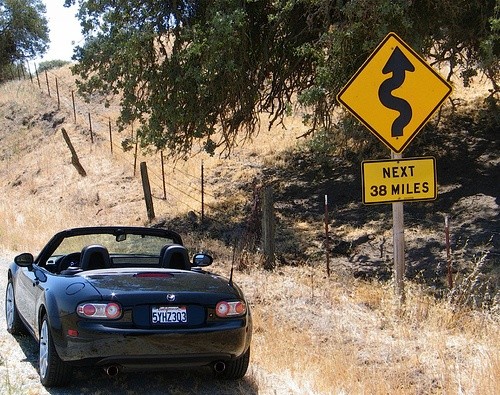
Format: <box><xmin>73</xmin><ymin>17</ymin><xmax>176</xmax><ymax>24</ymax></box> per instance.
<box><xmin>79</xmin><ymin>245</ymin><xmax>113</xmax><ymax>270</ymax></box>
<box><xmin>158</xmin><ymin>244</ymin><xmax>190</xmax><ymax>270</ymax></box>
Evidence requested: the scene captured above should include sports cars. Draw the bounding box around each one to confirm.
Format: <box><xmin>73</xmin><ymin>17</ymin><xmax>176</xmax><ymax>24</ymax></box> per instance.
<box><xmin>5</xmin><ymin>226</ymin><xmax>254</xmax><ymax>389</ymax></box>
